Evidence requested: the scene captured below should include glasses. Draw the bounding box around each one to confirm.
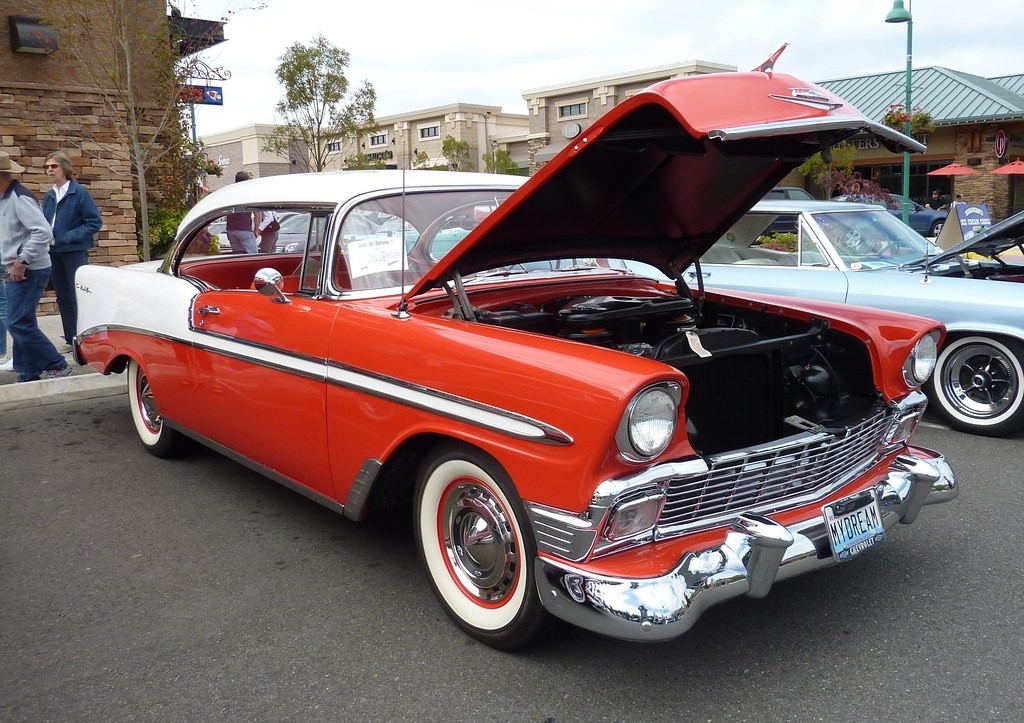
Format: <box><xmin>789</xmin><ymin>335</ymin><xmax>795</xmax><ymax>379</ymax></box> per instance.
<box><xmin>933</xmin><ymin>193</ymin><xmax>938</xmax><ymax>194</ymax></box>
<box><xmin>43</xmin><ymin>164</ymin><xmax>62</xmax><ymax>170</ymax></box>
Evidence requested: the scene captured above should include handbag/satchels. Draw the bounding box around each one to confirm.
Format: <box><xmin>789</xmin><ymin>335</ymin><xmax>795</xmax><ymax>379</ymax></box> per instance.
<box><xmin>261</xmin><ymin>218</ymin><xmax>280</xmax><ymax>235</ymax></box>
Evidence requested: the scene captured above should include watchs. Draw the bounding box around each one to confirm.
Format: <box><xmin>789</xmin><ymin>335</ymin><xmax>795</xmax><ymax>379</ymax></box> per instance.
<box><xmin>17</xmin><ymin>256</ymin><xmax>29</xmax><ymax>265</ymax></box>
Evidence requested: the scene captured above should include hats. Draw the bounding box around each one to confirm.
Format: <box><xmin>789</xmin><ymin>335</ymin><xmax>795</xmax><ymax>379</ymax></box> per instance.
<box><xmin>0</xmin><ymin>151</ymin><xmax>25</xmax><ymax>173</ymax></box>
<box><xmin>956</xmin><ymin>195</ymin><xmax>961</xmax><ymax>198</ymax></box>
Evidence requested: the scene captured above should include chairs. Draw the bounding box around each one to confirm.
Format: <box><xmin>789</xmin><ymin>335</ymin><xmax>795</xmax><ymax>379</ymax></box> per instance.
<box><xmin>778</xmin><ymin>251</ymin><xmax>823</xmax><ymax>266</ymax></box>
<box><xmin>250</xmin><ymin>270</ymin><xmax>343</xmax><ymax>293</ymax></box>
<box><xmin>732</xmin><ymin>258</ymin><xmax>780</xmax><ymax>266</ymax></box>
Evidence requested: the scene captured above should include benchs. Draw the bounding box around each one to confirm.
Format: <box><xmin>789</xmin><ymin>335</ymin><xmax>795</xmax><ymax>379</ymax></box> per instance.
<box><xmin>179</xmin><ymin>253</ymin><xmax>349</xmax><ymax>290</ymax></box>
<box><xmin>699</xmin><ymin>246</ymin><xmax>742</xmax><ymax>264</ymax></box>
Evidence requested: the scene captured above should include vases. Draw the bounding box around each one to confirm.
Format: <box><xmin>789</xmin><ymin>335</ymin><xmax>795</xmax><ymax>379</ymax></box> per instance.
<box><xmin>907</xmin><ymin>130</ymin><xmax>930</xmax><ymax>154</ymax></box>
<box><xmin>877</xmin><ymin>134</ymin><xmax>906</xmax><ymax>154</ymax></box>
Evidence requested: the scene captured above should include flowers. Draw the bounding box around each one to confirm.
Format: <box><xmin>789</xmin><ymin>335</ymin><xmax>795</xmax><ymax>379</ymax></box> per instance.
<box><xmin>910</xmin><ymin>106</ymin><xmax>935</xmax><ymax>131</ymax></box>
<box><xmin>880</xmin><ymin>103</ymin><xmax>909</xmax><ymax>128</ymax></box>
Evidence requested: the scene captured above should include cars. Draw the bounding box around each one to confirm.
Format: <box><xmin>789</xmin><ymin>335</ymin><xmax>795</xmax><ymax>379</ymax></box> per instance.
<box><xmin>362</xmin><ymin>211</ymin><xmax>399</xmax><ymax>225</ymax></box>
<box><xmin>255</xmin><ymin>212</ymin><xmax>379</xmax><ymax>255</ymax></box>
<box><xmin>678</xmin><ymin>199</ymin><xmax>1024</xmax><ymax>434</ymax></box>
<box><xmin>208</xmin><ymin>222</ymin><xmax>227</xmax><ymax>236</ymax></box>
<box><xmin>215</xmin><ymin>213</ymin><xmax>301</xmax><ymax>254</ymax></box>
<box><xmin>370</xmin><ymin>216</ymin><xmax>471</xmax><ymax>262</ymax></box>
<box><xmin>751</xmin><ymin>187</ymin><xmax>819</xmax><ymax>245</ymax></box>
<box><xmin>824</xmin><ymin>192</ymin><xmax>949</xmax><ymax>238</ymax></box>
<box><xmin>69</xmin><ymin>39</ymin><xmax>961</xmax><ymax>656</ymax></box>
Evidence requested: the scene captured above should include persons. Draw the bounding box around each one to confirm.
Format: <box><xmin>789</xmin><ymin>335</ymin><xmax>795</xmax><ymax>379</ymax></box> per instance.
<box><xmin>0</xmin><ymin>151</ymin><xmax>75</xmax><ymax>384</ymax></box>
<box><xmin>226</xmin><ymin>171</ymin><xmax>279</xmax><ymax>254</ymax></box>
<box><xmin>950</xmin><ymin>194</ymin><xmax>966</xmax><ymax>208</ymax></box>
<box><xmin>42</xmin><ymin>152</ymin><xmax>103</xmax><ymax>354</ymax></box>
<box><xmin>831</xmin><ymin>184</ymin><xmax>843</xmax><ymax>198</ymax></box>
<box><xmin>925</xmin><ymin>191</ymin><xmax>948</xmax><ymax>212</ymax></box>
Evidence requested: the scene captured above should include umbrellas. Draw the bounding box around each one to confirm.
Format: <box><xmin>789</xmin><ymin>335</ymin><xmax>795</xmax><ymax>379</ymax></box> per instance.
<box><xmin>927</xmin><ymin>161</ymin><xmax>981</xmax><ymax>192</ymax></box>
<box><xmin>989</xmin><ymin>157</ymin><xmax>1024</xmax><ymax>175</ymax></box>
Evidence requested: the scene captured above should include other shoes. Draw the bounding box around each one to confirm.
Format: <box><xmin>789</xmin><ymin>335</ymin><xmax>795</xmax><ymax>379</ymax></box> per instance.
<box><xmin>57</xmin><ymin>344</ymin><xmax>73</xmax><ymax>354</ymax></box>
<box><xmin>46</xmin><ymin>365</ymin><xmax>76</xmax><ymax>379</ymax></box>
<box><xmin>0</xmin><ymin>354</ymin><xmax>15</xmax><ymax>371</ymax></box>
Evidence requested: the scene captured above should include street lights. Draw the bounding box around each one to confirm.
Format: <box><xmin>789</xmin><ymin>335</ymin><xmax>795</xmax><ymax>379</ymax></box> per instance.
<box><xmin>885</xmin><ymin>1</ymin><xmax>913</xmax><ymax>227</ymax></box>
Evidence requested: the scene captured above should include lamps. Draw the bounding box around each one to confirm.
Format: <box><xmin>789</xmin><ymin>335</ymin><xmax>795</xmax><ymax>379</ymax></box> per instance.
<box><xmin>8</xmin><ymin>15</ymin><xmax>59</xmax><ymax>55</ymax></box>
